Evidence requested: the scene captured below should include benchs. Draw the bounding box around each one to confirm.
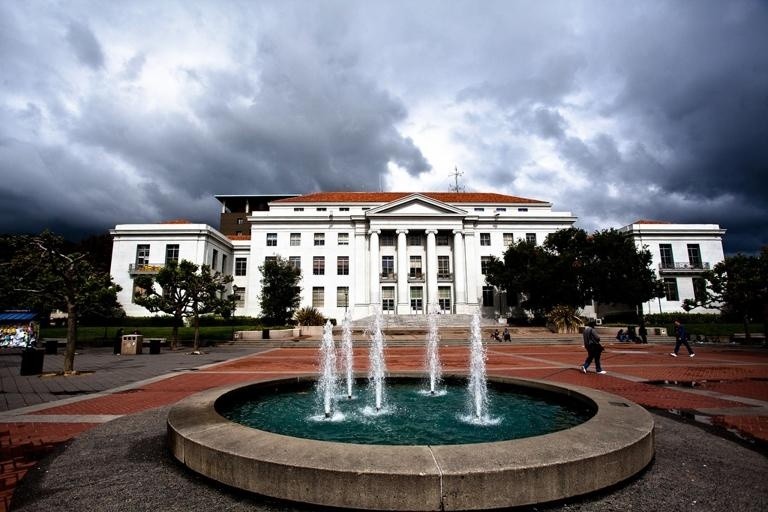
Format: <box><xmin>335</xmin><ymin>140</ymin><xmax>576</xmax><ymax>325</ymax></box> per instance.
<box><xmin>143</xmin><ymin>337</ymin><xmax>171</xmax><ymax>349</ymax></box>
<box><xmin>729</xmin><ymin>332</ymin><xmax>765</xmax><ymax>345</ymax></box>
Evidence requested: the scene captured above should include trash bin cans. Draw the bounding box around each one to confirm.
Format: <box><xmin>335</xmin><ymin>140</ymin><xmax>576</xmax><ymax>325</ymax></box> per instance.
<box><xmin>46</xmin><ymin>341</ymin><xmax>57</xmax><ymax>355</ymax></box>
<box><xmin>655</xmin><ymin>329</ymin><xmax>660</xmax><ymax>334</ymax></box>
<box><xmin>121</xmin><ymin>335</ymin><xmax>143</xmax><ymax>354</ymax></box>
<box><xmin>263</xmin><ymin>329</ymin><xmax>269</xmax><ymax>338</ymax></box>
<box><xmin>21</xmin><ymin>351</ymin><xmax>44</xmax><ymax>376</ymax></box>
<box><xmin>150</xmin><ymin>340</ymin><xmax>161</xmax><ymax>354</ymax></box>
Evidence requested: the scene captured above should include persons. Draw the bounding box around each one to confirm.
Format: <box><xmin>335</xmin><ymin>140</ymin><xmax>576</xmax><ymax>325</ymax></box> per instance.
<box><xmin>627</xmin><ymin>325</ymin><xmax>641</xmax><ymax>344</ymax></box>
<box><xmin>491</xmin><ymin>328</ymin><xmax>502</xmax><ymax>342</ymax></box>
<box><xmin>580</xmin><ymin>319</ymin><xmax>607</xmax><ymax>374</ymax></box>
<box><xmin>503</xmin><ymin>327</ymin><xmax>511</xmax><ymax>342</ymax></box>
<box><xmin>616</xmin><ymin>328</ymin><xmax>631</xmax><ymax>342</ymax></box>
<box><xmin>639</xmin><ymin>321</ymin><xmax>648</xmax><ymax>344</ymax></box>
<box><xmin>669</xmin><ymin>318</ymin><xmax>696</xmax><ymax>357</ymax></box>
<box><xmin>113</xmin><ymin>327</ymin><xmax>125</xmax><ymax>356</ymax></box>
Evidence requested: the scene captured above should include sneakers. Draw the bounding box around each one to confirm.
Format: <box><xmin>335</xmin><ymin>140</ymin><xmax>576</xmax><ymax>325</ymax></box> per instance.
<box><xmin>597</xmin><ymin>370</ymin><xmax>607</xmax><ymax>375</ymax></box>
<box><xmin>581</xmin><ymin>366</ymin><xmax>586</xmax><ymax>374</ymax></box>
<box><xmin>689</xmin><ymin>354</ymin><xmax>695</xmax><ymax>358</ymax></box>
<box><xmin>671</xmin><ymin>352</ymin><xmax>677</xmax><ymax>357</ymax></box>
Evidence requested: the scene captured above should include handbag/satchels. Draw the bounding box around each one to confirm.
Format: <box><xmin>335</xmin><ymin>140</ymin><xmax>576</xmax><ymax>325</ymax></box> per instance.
<box><xmin>587</xmin><ymin>343</ymin><xmax>603</xmax><ymax>355</ymax></box>
